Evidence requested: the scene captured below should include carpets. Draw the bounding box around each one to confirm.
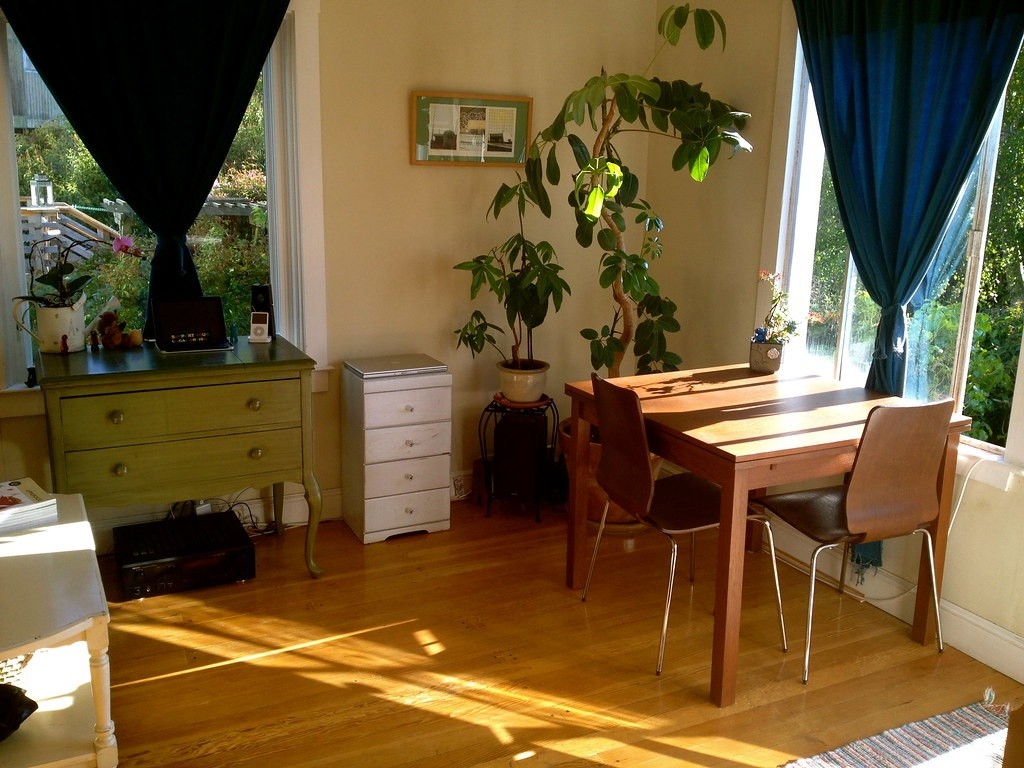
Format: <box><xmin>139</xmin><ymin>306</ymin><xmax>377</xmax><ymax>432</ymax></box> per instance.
<box><xmin>784</xmin><ymin>686</ymin><xmax>1010</xmax><ymax>768</ymax></box>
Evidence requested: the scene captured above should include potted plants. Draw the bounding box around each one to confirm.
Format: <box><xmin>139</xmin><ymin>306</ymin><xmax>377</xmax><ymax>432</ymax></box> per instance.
<box><xmin>551</xmin><ymin>0</ymin><xmax>753</xmax><ymax>524</ymax></box>
<box><xmin>452</xmin><ymin>130</ymin><xmax>571</xmax><ymax>403</ymax></box>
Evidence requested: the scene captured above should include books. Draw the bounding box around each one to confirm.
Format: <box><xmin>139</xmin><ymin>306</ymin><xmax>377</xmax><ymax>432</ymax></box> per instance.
<box><xmin>0</xmin><ymin>477</ymin><xmax>58</xmax><ymax>534</ymax></box>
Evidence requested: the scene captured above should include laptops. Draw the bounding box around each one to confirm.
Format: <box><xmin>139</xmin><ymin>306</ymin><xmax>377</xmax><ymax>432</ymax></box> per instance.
<box><xmin>151</xmin><ymin>296</ymin><xmax>234</xmax><ymax>354</ymax></box>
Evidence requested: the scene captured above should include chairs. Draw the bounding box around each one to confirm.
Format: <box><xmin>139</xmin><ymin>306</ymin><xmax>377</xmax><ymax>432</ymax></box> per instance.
<box><xmin>752</xmin><ymin>398</ymin><xmax>956</xmax><ymax>684</ymax></box>
<box><xmin>582</xmin><ymin>372</ymin><xmax>788</xmax><ymax>676</ymax></box>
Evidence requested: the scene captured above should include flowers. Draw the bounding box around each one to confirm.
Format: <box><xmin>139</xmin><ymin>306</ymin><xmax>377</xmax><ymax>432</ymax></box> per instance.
<box><xmin>11</xmin><ymin>234</ymin><xmax>148</xmax><ymax>342</ymax></box>
<box><xmin>750</xmin><ymin>269</ymin><xmax>800</xmax><ymax>349</ymax></box>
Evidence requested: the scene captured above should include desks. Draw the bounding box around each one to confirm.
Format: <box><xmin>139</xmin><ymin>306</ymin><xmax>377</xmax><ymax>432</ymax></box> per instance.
<box><xmin>34</xmin><ymin>334</ymin><xmax>324</xmax><ymax>579</ymax></box>
<box><xmin>478</xmin><ymin>392</ymin><xmax>559</xmax><ymax>523</ymax></box>
<box><xmin>564</xmin><ymin>361</ymin><xmax>972</xmax><ymax>708</ymax></box>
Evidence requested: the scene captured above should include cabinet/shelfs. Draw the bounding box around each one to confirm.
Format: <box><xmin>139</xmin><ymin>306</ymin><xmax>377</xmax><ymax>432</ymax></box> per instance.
<box><xmin>341</xmin><ymin>367</ymin><xmax>452</xmax><ymax>544</ymax></box>
<box><xmin>0</xmin><ymin>494</ymin><xmax>118</xmax><ymax>768</ymax></box>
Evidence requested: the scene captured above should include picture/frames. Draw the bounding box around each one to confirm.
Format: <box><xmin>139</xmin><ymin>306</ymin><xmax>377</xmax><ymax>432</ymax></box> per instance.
<box><xmin>409</xmin><ymin>90</ymin><xmax>533</xmax><ymax>167</ymax></box>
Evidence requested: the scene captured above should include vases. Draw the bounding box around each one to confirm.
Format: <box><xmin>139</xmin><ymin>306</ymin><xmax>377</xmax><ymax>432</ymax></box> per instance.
<box><xmin>35</xmin><ymin>290</ymin><xmax>87</xmax><ymax>353</ymax></box>
<box><xmin>750</xmin><ymin>343</ymin><xmax>782</xmax><ymax>372</ymax></box>
